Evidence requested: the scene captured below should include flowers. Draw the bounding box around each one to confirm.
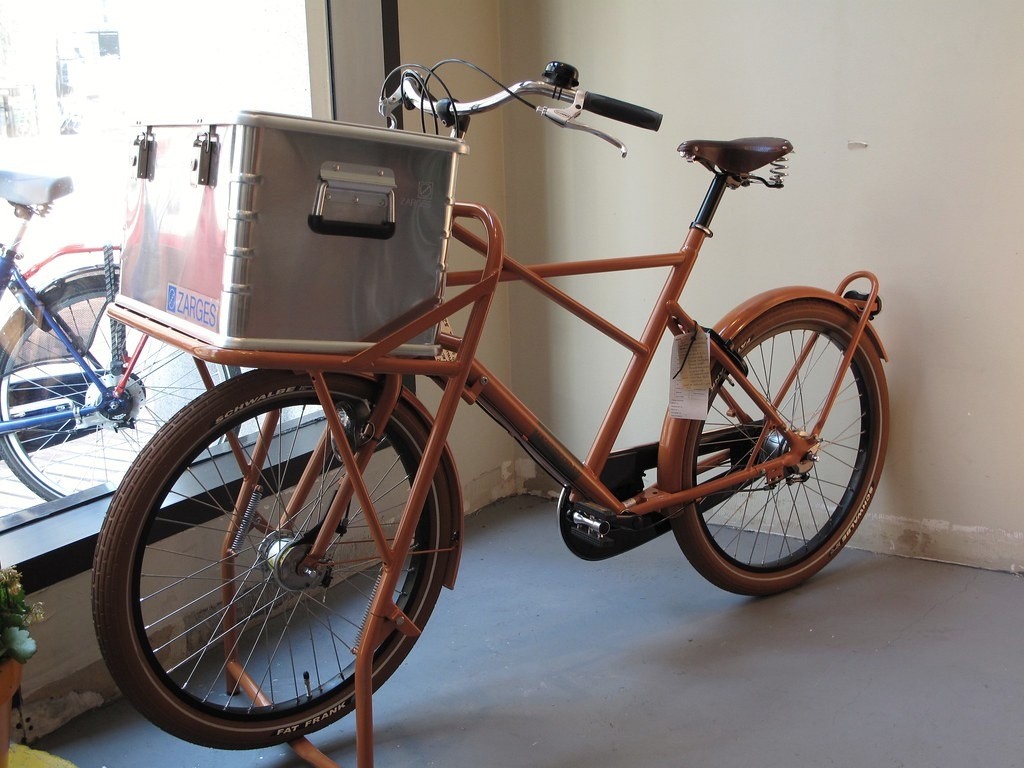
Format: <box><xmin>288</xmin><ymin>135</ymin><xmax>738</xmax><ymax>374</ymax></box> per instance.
<box><xmin>0</xmin><ymin>561</ymin><xmax>46</xmax><ymax>666</ymax></box>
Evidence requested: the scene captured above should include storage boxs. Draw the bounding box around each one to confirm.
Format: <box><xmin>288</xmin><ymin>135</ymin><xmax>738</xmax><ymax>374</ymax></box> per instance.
<box><xmin>112</xmin><ymin>106</ymin><xmax>474</xmax><ymax>357</ymax></box>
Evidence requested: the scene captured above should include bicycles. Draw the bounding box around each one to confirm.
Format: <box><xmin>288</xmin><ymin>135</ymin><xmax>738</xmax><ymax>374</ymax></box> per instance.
<box><xmin>89</xmin><ymin>56</ymin><xmax>894</xmax><ymax>768</ymax></box>
<box><xmin>0</xmin><ymin>170</ymin><xmax>244</xmax><ymax>503</ymax></box>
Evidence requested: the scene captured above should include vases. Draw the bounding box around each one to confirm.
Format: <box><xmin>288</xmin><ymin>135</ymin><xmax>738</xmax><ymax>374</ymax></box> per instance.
<box><xmin>0</xmin><ymin>657</ymin><xmax>26</xmax><ymax>767</ymax></box>
